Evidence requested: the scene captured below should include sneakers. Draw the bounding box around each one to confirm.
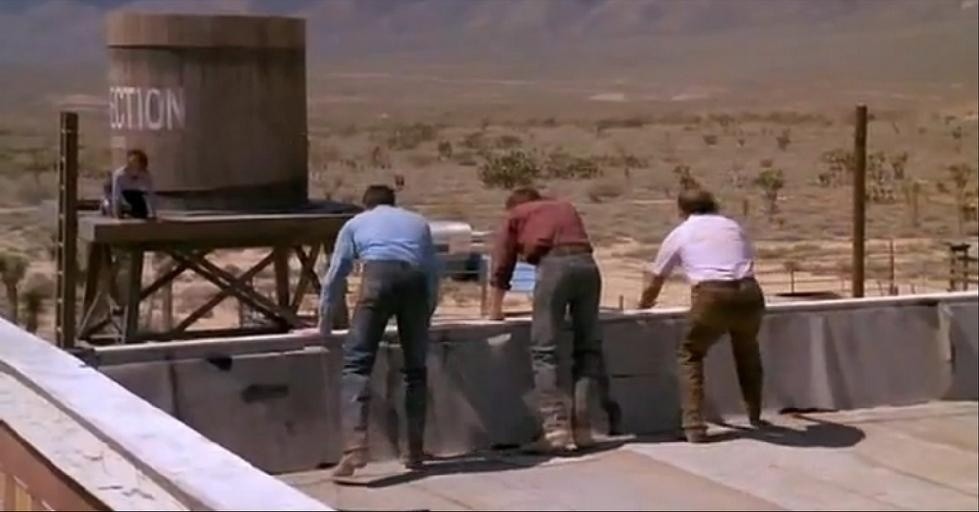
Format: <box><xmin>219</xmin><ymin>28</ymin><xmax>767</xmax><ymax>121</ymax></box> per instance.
<box><xmin>334</xmin><ymin>449</ymin><xmax>367</xmax><ymax>475</ymax></box>
<box><xmin>405</xmin><ymin>457</ymin><xmax>424</xmax><ymax>469</ymax></box>
<box><xmin>520</xmin><ymin>424</ymin><xmax>610</xmax><ymax>452</ymax></box>
<box><xmin>675</xmin><ymin>430</ymin><xmax>708</xmax><ymax>443</ymax></box>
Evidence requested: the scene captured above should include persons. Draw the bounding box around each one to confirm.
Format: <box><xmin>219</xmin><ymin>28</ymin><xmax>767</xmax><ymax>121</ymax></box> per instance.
<box><xmin>635</xmin><ymin>187</ymin><xmax>764</xmax><ymax>442</ymax></box>
<box><xmin>485</xmin><ymin>185</ymin><xmax>604</xmax><ymax>454</ymax></box>
<box><xmin>100</xmin><ymin>148</ymin><xmax>159</xmax><ymax>223</ymax></box>
<box><xmin>289</xmin><ymin>185</ymin><xmax>440</xmax><ymax>478</ymax></box>
